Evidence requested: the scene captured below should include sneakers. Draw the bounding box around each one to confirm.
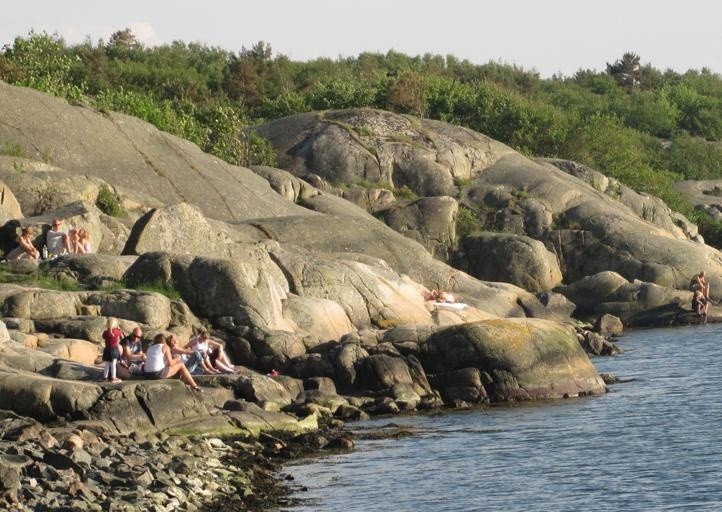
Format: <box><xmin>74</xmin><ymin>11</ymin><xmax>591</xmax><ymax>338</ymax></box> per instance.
<box><xmin>192</xmin><ymin>385</ymin><xmax>204</xmax><ymax>392</ymax></box>
<box><xmin>111</xmin><ymin>378</ymin><xmax>122</xmax><ymax>383</ymax></box>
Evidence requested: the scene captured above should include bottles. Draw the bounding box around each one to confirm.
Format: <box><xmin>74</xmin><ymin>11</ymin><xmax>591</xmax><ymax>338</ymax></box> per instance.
<box><xmin>42</xmin><ymin>243</ymin><xmax>48</xmax><ymax>259</ymax></box>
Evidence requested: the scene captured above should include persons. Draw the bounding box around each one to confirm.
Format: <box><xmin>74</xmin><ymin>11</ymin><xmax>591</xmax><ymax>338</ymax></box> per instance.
<box><xmin>100</xmin><ymin>315</ymin><xmax>242</xmax><ymax>394</ymax></box>
<box><xmin>1</xmin><ymin>219</ymin><xmax>92</xmax><ymax>263</ymax></box>
<box><xmin>688</xmin><ymin>270</ymin><xmax>710</xmax><ymax>326</ymax></box>
